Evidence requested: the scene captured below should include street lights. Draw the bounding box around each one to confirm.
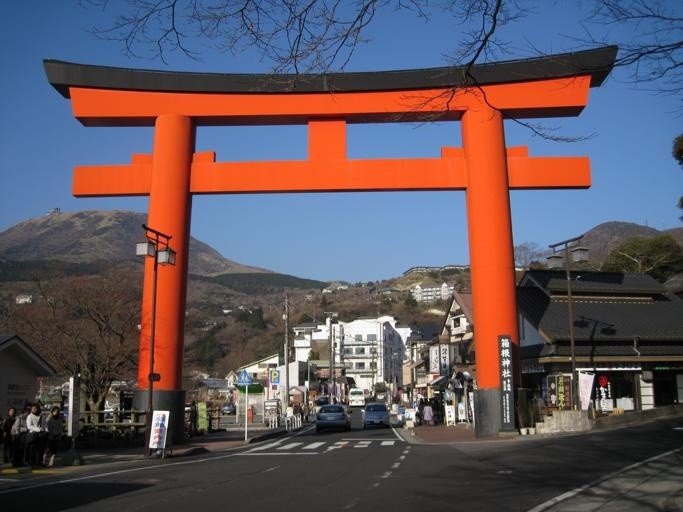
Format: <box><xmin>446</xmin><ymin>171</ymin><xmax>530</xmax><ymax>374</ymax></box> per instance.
<box><xmin>135</xmin><ymin>223</ymin><xmax>176</xmax><ymax>457</ymax></box>
<box><xmin>546</xmin><ymin>233</ymin><xmax>589</xmax><ymax>409</ymax></box>
<box><xmin>390</xmin><ymin>348</ymin><xmax>397</xmax><ymax>397</ymax></box>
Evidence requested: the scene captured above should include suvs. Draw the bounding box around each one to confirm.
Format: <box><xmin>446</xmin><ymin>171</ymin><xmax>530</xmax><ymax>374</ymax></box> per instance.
<box><xmin>220</xmin><ymin>402</ymin><xmax>235</xmax><ymax>415</ymax></box>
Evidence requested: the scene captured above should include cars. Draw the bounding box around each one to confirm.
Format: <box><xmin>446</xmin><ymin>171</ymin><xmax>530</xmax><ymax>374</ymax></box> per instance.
<box><xmin>312</xmin><ymin>386</ymin><xmax>391</xmax><ymax>434</ymax></box>
<box><xmin>184</xmin><ymin>403</ymin><xmax>194</xmax><ymax>412</ymax></box>
<box><xmin>46</xmin><ymin>387</ymin><xmax>60</xmax><ymax>397</ymax></box>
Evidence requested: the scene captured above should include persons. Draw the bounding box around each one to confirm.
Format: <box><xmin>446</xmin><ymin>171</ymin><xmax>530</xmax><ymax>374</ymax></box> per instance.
<box><xmin>0</xmin><ymin>401</ymin><xmax>65</xmax><ymax>469</ymax></box>
<box><xmin>416</xmin><ymin>398</ymin><xmax>442</xmax><ymax>426</ymax></box>
<box><xmin>284</xmin><ymin>401</ymin><xmax>306</xmax><ymax>422</ymax></box>
